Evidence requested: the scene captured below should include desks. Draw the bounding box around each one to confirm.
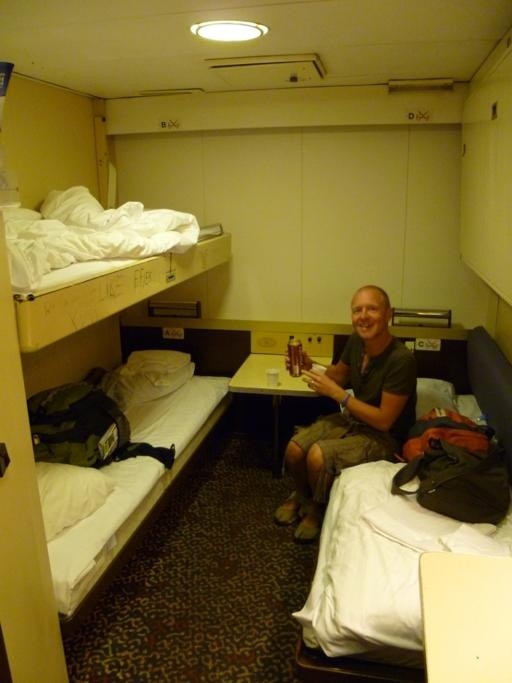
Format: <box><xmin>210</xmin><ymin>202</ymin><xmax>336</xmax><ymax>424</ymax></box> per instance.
<box><xmin>227</xmin><ymin>351</ymin><xmax>332</xmax><ymax>479</ymax></box>
<box><xmin>417</xmin><ymin>550</ymin><xmax>512</xmax><ymax>682</ymax></box>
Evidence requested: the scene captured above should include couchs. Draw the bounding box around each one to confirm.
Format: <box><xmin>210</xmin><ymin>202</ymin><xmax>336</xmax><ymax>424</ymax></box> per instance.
<box><xmin>294</xmin><ymin>325</ymin><xmax>512</xmax><ymax>682</ymax></box>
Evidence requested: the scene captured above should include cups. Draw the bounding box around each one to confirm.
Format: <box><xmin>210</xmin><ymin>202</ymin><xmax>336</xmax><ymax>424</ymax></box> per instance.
<box><xmin>265</xmin><ymin>368</ymin><xmax>280</xmax><ymax>387</ymax></box>
<box><xmin>311</xmin><ymin>363</ymin><xmax>327</xmax><ymax>374</ymax></box>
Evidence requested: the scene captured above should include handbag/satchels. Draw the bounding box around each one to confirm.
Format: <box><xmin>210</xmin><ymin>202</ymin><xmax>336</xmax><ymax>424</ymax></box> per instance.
<box><xmin>403</xmin><ymin>407</ymin><xmax>495</xmax><ymax>464</ymax></box>
<box><xmin>392</xmin><ymin>439</ymin><xmax>511</xmax><ymax>524</ymax></box>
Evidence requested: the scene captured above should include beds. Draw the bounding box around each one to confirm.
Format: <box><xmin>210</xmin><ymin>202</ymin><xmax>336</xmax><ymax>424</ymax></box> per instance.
<box><xmin>0</xmin><ymin>197</ymin><xmax>235</xmax><ymax>682</ymax></box>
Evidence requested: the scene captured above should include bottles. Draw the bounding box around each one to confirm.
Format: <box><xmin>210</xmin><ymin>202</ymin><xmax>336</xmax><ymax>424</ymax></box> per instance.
<box><xmin>478</xmin><ymin>415</ymin><xmax>487</xmax><ymax>425</ymax></box>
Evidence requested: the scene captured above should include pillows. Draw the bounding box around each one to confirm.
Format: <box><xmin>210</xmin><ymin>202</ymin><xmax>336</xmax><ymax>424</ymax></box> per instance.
<box><xmin>33</xmin><ymin>458</ymin><xmax>115</xmax><ymax>539</ymax></box>
<box><xmin>111</xmin><ymin>361</ymin><xmax>195</xmax><ymax>402</ymax></box>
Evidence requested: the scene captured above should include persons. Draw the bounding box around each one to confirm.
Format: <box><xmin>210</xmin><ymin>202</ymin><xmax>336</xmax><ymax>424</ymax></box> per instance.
<box><xmin>275</xmin><ymin>286</ymin><xmax>417</xmax><ymax>542</ymax></box>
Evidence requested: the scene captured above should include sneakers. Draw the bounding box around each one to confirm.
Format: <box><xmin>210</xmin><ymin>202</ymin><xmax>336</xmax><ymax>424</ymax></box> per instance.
<box><xmin>294</xmin><ymin>505</ymin><xmax>324</xmax><ymax>540</ymax></box>
<box><xmin>274</xmin><ymin>490</ymin><xmax>304</xmax><ymax>523</ymax></box>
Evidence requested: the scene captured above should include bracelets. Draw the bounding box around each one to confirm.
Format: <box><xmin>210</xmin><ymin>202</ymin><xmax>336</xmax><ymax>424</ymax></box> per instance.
<box><xmin>342</xmin><ymin>394</ymin><xmax>351</xmax><ymax>407</ymax></box>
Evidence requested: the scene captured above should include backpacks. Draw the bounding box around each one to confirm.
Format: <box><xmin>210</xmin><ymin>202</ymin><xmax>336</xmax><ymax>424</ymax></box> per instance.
<box><xmin>26</xmin><ymin>380</ymin><xmax>175</xmax><ymax>469</ymax></box>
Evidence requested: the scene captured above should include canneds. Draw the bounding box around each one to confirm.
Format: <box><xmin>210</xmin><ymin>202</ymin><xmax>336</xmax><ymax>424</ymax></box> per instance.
<box><xmin>287</xmin><ymin>339</ymin><xmax>304</xmax><ymax>377</ymax></box>
